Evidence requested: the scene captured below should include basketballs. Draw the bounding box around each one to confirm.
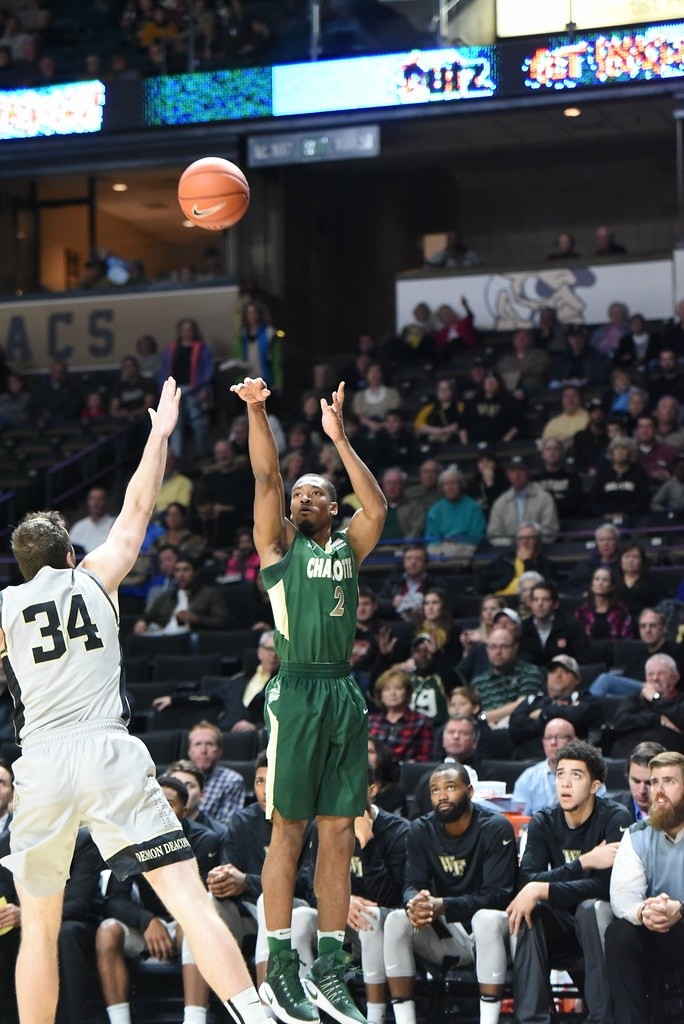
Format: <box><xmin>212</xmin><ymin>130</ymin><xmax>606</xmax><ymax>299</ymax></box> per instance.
<box><xmin>177</xmin><ymin>156</ymin><xmax>250</xmax><ymax>231</ymax></box>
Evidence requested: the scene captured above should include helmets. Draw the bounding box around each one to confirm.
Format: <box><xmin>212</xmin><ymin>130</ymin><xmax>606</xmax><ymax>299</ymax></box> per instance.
<box><xmin>438</xmin><ymin>472</ymin><xmax>464</xmax><ymax>490</ymax></box>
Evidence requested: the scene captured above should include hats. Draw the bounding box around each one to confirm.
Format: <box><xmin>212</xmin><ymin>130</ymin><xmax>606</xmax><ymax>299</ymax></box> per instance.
<box><xmin>493</xmin><ymin>607</ymin><xmax>522</xmax><ymax>628</ymax></box>
<box><xmin>546</xmin><ymin>654</ymin><xmax>581</xmax><ymax>679</ymax></box>
<box><xmin>507</xmin><ymin>455</ymin><xmax>528</xmax><ymax>472</ymax></box>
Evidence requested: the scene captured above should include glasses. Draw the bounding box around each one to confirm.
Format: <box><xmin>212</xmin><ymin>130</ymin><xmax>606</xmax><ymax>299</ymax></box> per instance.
<box><xmin>541</xmin><ymin>734</ymin><xmax>573</xmax><ymax>744</ymax></box>
<box><xmin>260</xmin><ymin>643</ymin><xmax>277</xmax><ymax>656</ymax></box>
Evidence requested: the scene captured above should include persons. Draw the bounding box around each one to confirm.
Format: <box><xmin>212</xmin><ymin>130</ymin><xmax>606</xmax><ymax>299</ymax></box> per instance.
<box><xmin>0</xmin><ymin>376</ymin><xmax>277</xmax><ymax>1024</ymax></box>
<box><xmin>0</xmin><ymin>227</ymin><xmax>684</xmax><ymax>1024</ymax></box>
<box><xmin>230</xmin><ymin>376</ymin><xmax>387</xmax><ymax>1024</ymax></box>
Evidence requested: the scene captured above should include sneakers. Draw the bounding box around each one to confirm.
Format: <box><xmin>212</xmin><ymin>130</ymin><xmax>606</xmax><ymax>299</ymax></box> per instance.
<box><xmin>302</xmin><ymin>954</ymin><xmax>368</xmax><ymax>1024</ymax></box>
<box><xmin>258</xmin><ymin>948</ymin><xmax>321</xmax><ymax>1024</ymax></box>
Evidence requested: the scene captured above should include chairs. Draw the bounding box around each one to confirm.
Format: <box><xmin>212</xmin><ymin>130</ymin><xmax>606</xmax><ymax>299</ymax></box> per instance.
<box><xmin>0</xmin><ymin>316</ymin><xmax>683</xmax><ymax>1024</ymax></box>
